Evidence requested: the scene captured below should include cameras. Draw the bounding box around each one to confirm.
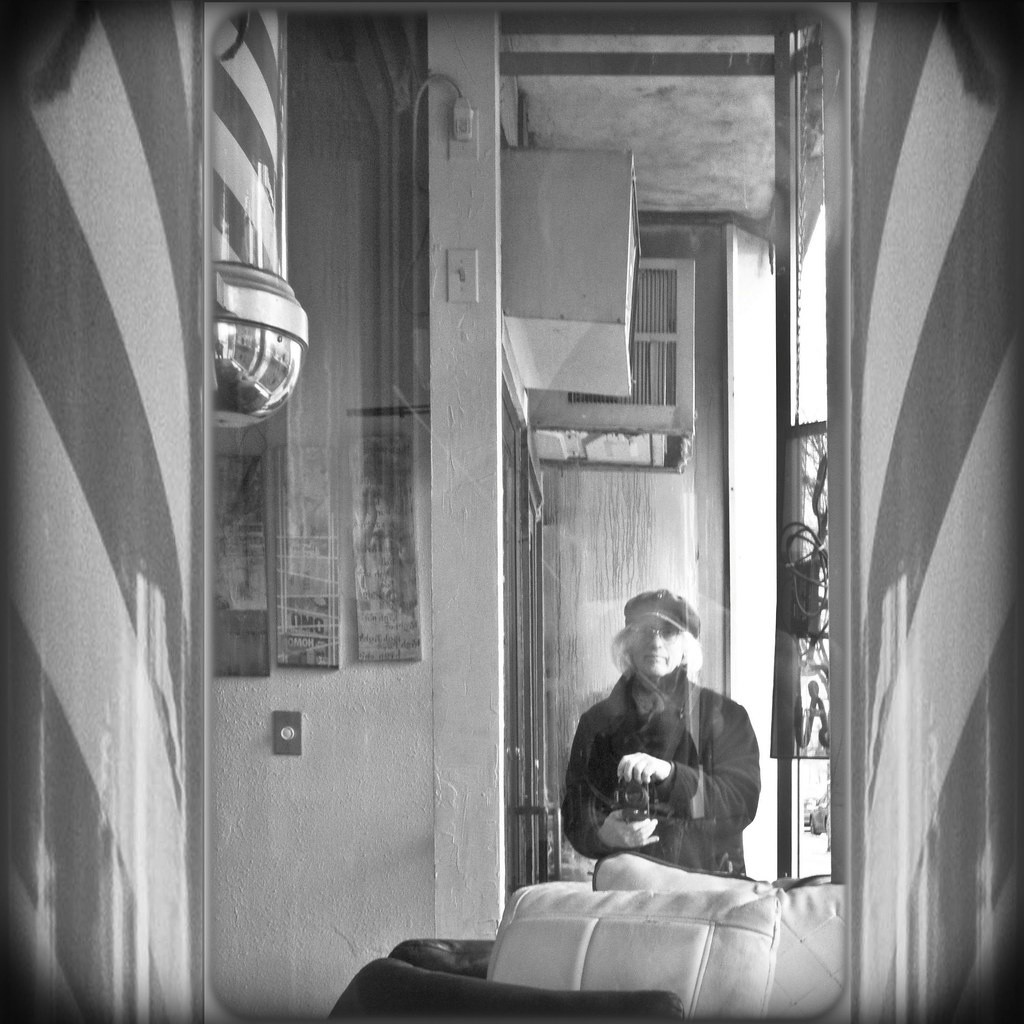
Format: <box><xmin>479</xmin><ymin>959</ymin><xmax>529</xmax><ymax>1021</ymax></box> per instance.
<box><xmin>612</xmin><ymin>775</ymin><xmax>650</xmax><ymax>822</ymax></box>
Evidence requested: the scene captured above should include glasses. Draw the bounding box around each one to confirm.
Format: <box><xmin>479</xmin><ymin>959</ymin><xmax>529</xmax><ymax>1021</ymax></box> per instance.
<box><xmin>633</xmin><ymin>623</ymin><xmax>678</xmax><ymax>645</ymax></box>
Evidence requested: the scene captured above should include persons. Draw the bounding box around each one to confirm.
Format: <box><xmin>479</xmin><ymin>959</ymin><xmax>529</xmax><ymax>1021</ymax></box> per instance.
<box><xmin>562</xmin><ymin>590</ymin><xmax>762</xmax><ymax>877</ymax></box>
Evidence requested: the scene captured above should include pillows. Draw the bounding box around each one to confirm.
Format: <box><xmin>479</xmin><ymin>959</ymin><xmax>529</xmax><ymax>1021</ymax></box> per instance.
<box><xmin>482</xmin><ymin>849</ymin><xmax>869</xmax><ymax>1024</ymax></box>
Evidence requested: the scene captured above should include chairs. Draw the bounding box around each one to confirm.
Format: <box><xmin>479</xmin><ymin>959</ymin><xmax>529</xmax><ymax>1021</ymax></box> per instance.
<box><xmin>321</xmin><ymin>937</ymin><xmax>792</xmax><ymax>1024</ymax></box>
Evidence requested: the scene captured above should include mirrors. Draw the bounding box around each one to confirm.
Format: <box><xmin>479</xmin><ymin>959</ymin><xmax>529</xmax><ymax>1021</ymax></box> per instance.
<box><xmin>191</xmin><ymin>0</ymin><xmax>852</xmax><ymax>1024</ymax></box>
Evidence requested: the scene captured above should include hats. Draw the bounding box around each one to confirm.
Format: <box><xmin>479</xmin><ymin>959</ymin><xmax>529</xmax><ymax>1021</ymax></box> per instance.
<box><xmin>624</xmin><ymin>588</ymin><xmax>701</xmax><ymax>639</ymax></box>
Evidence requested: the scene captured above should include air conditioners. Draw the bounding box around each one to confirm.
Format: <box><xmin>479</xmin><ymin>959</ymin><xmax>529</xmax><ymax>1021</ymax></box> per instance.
<box><xmin>498</xmin><ymin>136</ymin><xmax>642</xmax><ymax>399</ymax></box>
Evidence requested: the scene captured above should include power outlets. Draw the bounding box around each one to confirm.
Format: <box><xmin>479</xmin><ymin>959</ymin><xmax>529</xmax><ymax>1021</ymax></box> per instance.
<box><xmin>447</xmin><ymin>104</ymin><xmax>482</xmax><ymax>165</ymax></box>
